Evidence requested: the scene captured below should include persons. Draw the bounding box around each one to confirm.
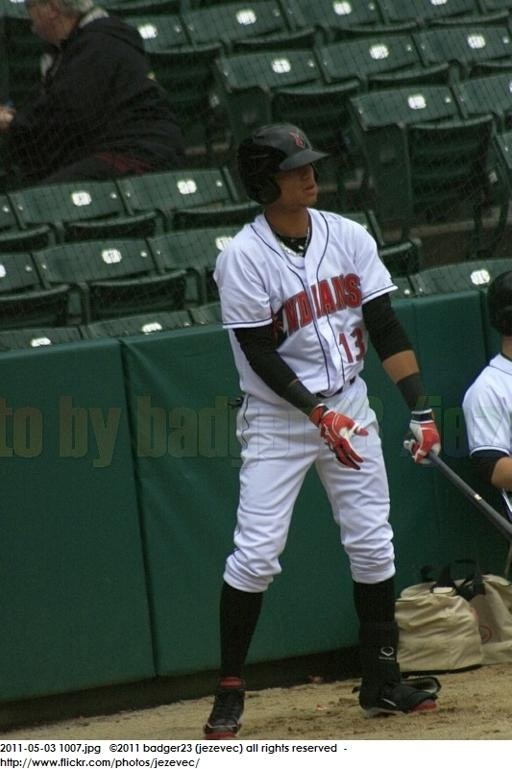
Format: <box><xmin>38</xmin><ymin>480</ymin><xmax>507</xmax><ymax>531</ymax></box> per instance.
<box><xmin>0</xmin><ymin>0</ymin><xmax>187</xmax><ymax>203</ymax></box>
<box><xmin>201</xmin><ymin>120</ymin><xmax>443</xmax><ymax>741</ymax></box>
<box><xmin>460</xmin><ymin>267</ymin><xmax>512</xmax><ymax>495</ymax></box>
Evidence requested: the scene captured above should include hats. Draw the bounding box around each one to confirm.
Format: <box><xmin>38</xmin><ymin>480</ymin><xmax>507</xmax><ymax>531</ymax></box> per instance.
<box><xmin>234</xmin><ymin>122</ymin><xmax>328</xmax><ymax>185</ymax></box>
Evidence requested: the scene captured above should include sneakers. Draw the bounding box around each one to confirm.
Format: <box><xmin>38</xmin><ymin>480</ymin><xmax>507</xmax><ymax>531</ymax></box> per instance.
<box><xmin>203</xmin><ymin>683</ymin><xmax>246</xmax><ymax>740</ymax></box>
<box><xmin>358</xmin><ymin>673</ymin><xmax>438</xmax><ymax>719</ymax></box>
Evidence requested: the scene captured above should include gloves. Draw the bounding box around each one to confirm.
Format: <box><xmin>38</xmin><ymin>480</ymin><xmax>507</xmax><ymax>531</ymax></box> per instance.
<box><xmin>308</xmin><ymin>403</ymin><xmax>368</xmax><ymax>471</ymax></box>
<box><xmin>403</xmin><ymin>409</ymin><xmax>442</xmax><ymax>465</ymax></box>
